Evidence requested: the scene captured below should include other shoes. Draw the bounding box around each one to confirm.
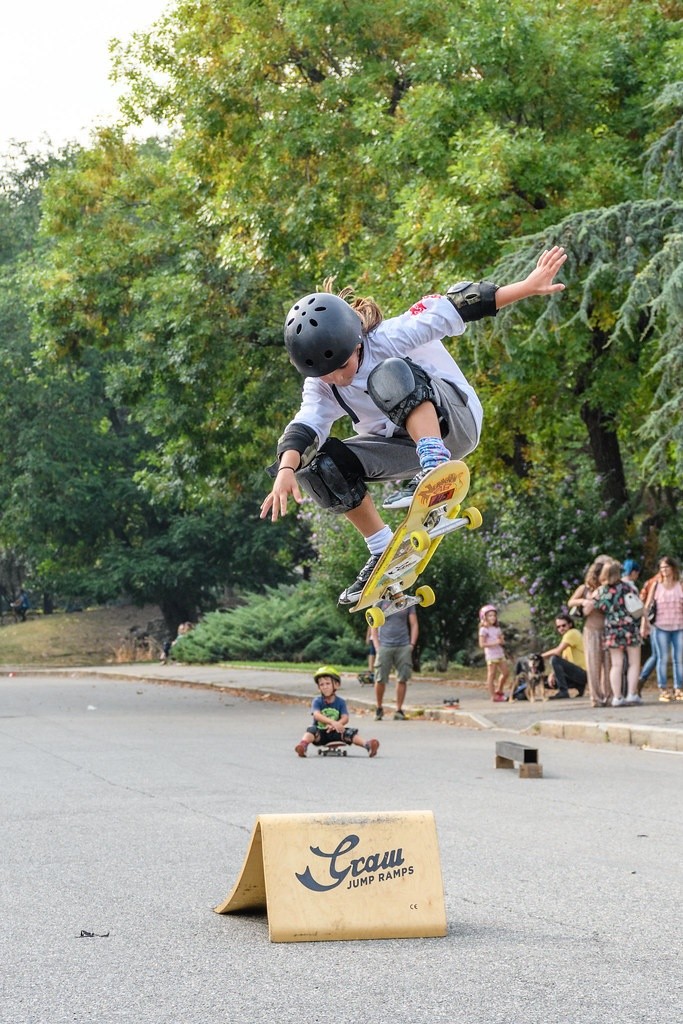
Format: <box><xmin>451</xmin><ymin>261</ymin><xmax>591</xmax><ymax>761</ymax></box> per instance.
<box><xmin>494</xmin><ymin>696</ymin><xmax>508</xmax><ymax>701</ymax></box>
<box><xmin>367</xmin><ymin>739</ymin><xmax>378</xmax><ymax>757</ymax></box>
<box><xmin>296</xmin><ymin>744</ymin><xmax>307</xmax><ymax>757</ymax></box>
<box><xmin>659</xmin><ymin>691</ymin><xmax>672</xmax><ymax>702</ymax></box>
<box><xmin>674</xmin><ymin>691</ymin><xmax>683</xmax><ymax>700</ymax></box>
<box><xmin>577</xmin><ymin>688</ymin><xmax>585</xmax><ymax>698</ymax></box>
<box><xmin>590</xmin><ymin>693</ymin><xmax>642</xmax><ymax>707</ymax></box>
<box><xmin>548</xmin><ymin>692</ymin><xmax>569</xmax><ymax>700</ymax></box>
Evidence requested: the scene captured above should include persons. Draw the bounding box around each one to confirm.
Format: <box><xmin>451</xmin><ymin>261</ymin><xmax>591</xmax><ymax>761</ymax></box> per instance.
<box><xmin>371</xmin><ymin>591</ymin><xmax>418</xmax><ymax>721</ymax></box>
<box><xmin>260</xmin><ymin>246</ymin><xmax>567</xmax><ymax>605</ymax></box>
<box><xmin>619</xmin><ymin>554</ymin><xmax>683</xmax><ymax>702</ymax></box>
<box><xmin>567</xmin><ymin>554</ymin><xmax>614</xmax><ymax>707</ymax></box>
<box><xmin>13</xmin><ymin>588</ymin><xmax>31</xmax><ymax>622</ymax></box>
<box><xmin>541</xmin><ymin>613</ymin><xmax>588</xmax><ymax>700</ymax></box>
<box><xmin>160</xmin><ymin>622</ymin><xmax>194</xmax><ymax>666</ymax></box>
<box><xmin>367</xmin><ymin>626</ymin><xmax>380</xmax><ymax>671</ymax></box>
<box><xmin>294</xmin><ymin>667</ymin><xmax>379</xmax><ymax>757</ymax></box>
<box><xmin>583</xmin><ymin>559</ymin><xmax>644</xmax><ymax>705</ymax></box>
<box><xmin>478</xmin><ymin>605</ymin><xmax>510</xmax><ymax>702</ymax></box>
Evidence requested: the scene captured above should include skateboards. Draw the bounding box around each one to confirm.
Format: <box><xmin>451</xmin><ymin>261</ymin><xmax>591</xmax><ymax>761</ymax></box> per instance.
<box><xmin>348</xmin><ymin>460</ymin><xmax>483</xmax><ymax>629</ymax></box>
<box><xmin>356</xmin><ymin>673</ymin><xmax>388</xmax><ymax>688</ymax></box>
<box><xmin>441</xmin><ymin>698</ymin><xmax>460</xmax><ymax>710</ymax></box>
<box><xmin>318</xmin><ymin>741</ymin><xmax>347</xmax><ymax>757</ymax></box>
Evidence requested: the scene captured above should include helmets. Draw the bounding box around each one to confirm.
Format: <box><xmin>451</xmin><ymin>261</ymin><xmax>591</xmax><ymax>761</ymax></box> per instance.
<box><xmin>315</xmin><ymin>666</ymin><xmax>341</xmax><ymax>683</ymax></box>
<box><xmin>282</xmin><ymin>292</ymin><xmax>362</xmax><ymax>376</ymax></box>
<box><xmin>480</xmin><ymin>605</ymin><xmax>497</xmax><ymax>623</ymax></box>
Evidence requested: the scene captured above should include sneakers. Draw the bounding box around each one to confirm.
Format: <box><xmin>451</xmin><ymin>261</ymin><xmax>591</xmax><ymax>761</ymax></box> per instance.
<box><xmin>393</xmin><ymin>711</ymin><xmax>411</xmax><ymax>720</ymax></box>
<box><xmin>337</xmin><ymin>553</ymin><xmax>383</xmax><ymax>605</ymax></box>
<box><xmin>376</xmin><ymin>708</ymin><xmax>384</xmax><ymax>720</ymax></box>
<box><xmin>380</xmin><ymin>474</ymin><xmax>418</xmax><ymax>509</ymax></box>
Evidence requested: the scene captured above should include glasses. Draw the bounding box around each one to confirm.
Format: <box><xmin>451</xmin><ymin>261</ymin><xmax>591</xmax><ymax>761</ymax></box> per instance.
<box><xmin>556</xmin><ymin>624</ymin><xmax>567</xmax><ymax>628</ymax></box>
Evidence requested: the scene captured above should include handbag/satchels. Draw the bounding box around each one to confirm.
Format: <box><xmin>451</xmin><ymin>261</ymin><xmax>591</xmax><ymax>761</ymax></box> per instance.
<box><xmin>644</xmin><ymin>581</ymin><xmax>659</xmax><ymax>625</ymax></box>
<box><xmin>569</xmin><ymin>586</ymin><xmax>588</xmax><ymax>617</ymax></box>
<box><xmin>622</xmin><ymin>584</ymin><xmax>645</xmax><ymax>619</ymax></box>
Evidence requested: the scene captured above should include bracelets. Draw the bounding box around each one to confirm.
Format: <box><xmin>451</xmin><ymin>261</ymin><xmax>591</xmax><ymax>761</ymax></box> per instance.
<box><xmin>410</xmin><ymin>643</ymin><xmax>415</xmax><ymax>647</ymax></box>
<box><xmin>278</xmin><ymin>466</ymin><xmax>294</xmax><ymax>471</ymax></box>
